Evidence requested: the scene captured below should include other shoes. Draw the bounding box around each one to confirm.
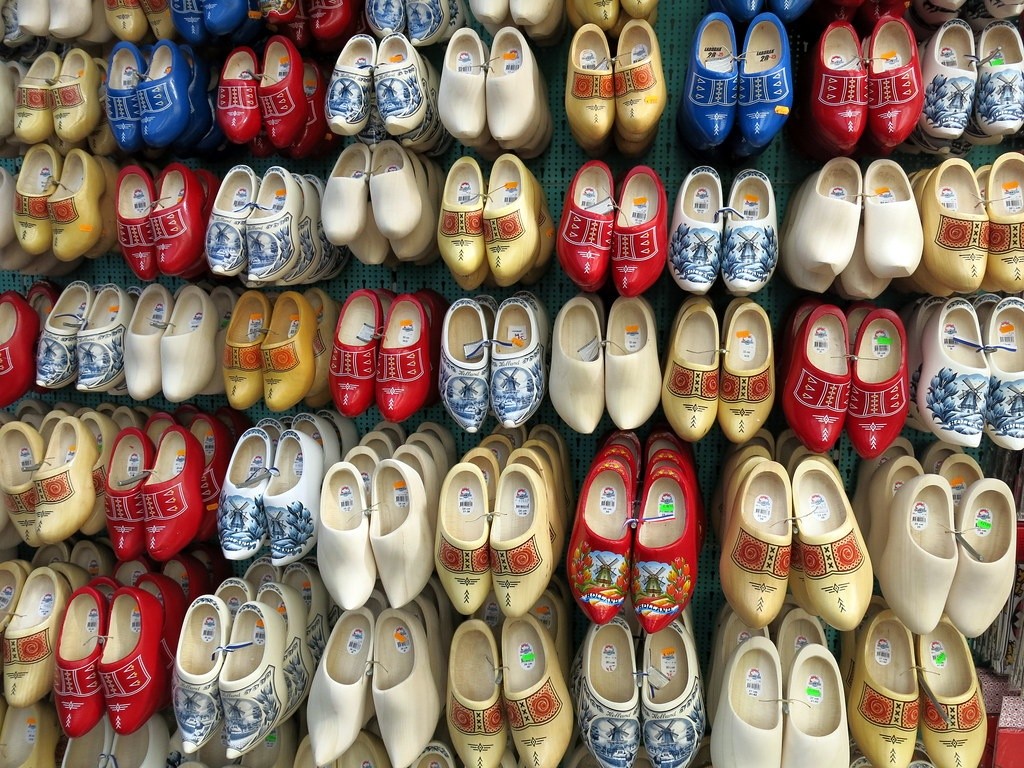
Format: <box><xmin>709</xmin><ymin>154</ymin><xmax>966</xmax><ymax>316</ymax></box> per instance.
<box><xmin>0</xmin><ymin>0</ymin><xmax>1024</xmax><ymax>767</ymax></box>
